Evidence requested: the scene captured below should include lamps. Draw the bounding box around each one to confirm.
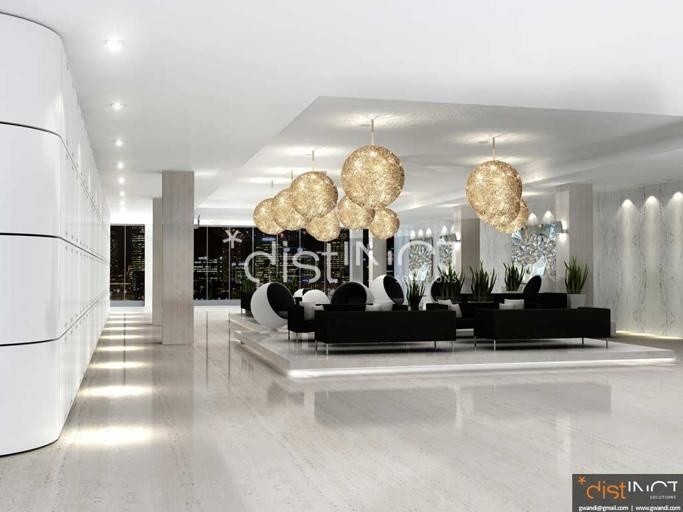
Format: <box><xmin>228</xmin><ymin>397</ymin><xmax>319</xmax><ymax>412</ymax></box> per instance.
<box><xmin>253</xmin><ymin>118</ymin><xmax>405</xmax><ymax>244</ymax></box>
<box><xmin>465</xmin><ymin>137</ymin><xmax>530</xmax><ymax>235</ymax></box>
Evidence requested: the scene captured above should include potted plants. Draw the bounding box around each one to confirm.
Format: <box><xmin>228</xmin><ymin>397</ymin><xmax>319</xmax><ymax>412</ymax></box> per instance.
<box><xmin>404</xmin><ymin>255</ymin><xmax>590</xmax><ymax>310</ymax></box>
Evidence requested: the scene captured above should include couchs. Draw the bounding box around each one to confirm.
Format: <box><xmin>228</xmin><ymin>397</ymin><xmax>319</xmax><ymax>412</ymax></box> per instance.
<box><xmin>239</xmin><ymin>292</ymin><xmax>611</xmax><ymax>356</ymax></box>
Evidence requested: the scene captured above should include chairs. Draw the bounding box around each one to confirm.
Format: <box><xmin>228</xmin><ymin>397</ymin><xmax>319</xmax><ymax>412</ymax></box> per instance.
<box><xmin>250</xmin><ymin>274</ymin><xmax>462</xmax><ymax>334</ymax></box>
